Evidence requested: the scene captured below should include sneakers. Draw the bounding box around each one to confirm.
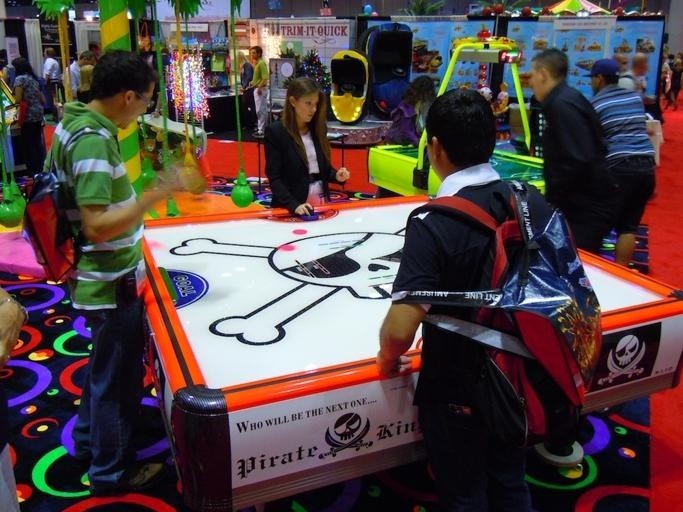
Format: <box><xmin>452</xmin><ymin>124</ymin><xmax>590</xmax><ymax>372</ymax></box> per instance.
<box><xmin>251</xmin><ymin>130</ymin><xmax>265</xmax><ymax>139</ymax></box>
<box><xmin>73</xmin><ymin>444</ymin><xmax>96</xmax><ymax>471</ymax></box>
<box><xmin>89</xmin><ymin>461</ymin><xmax>168</xmax><ymax>496</ymax></box>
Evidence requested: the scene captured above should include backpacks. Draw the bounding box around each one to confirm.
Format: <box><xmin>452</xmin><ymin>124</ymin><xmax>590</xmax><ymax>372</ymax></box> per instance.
<box><xmin>20</xmin><ymin>127</ymin><xmax>112</xmax><ymax>284</ymax></box>
<box><xmin>392</xmin><ymin>178</ymin><xmax>603</xmax><ymax>447</ymax></box>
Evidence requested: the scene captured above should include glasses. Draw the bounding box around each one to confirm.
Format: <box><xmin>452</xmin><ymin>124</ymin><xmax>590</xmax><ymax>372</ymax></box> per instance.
<box><xmin>133</xmin><ymin>90</ymin><xmax>155</xmax><ymax>109</ymax></box>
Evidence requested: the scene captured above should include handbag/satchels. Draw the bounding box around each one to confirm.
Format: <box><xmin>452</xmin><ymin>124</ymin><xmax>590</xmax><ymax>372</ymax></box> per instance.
<box><xmin>17</xmin><ymin>100</ymin><xmax>28</xmax><ymax>127</ymax></box>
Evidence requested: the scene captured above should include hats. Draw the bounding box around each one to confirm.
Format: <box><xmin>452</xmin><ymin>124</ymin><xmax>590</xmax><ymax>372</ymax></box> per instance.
<box><xmin>579</xmin><ymin>58</ymin><xmax>621</xmax><ymax>80</ymax></box>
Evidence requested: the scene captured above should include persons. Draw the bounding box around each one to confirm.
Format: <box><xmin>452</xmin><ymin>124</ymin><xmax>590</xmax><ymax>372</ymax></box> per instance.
<box><xmin>529</xmin><ymin>44</ymin><xmax>606</xmax><ymax>257</ymax></box>
<box><xmin>618</xmin><ymin>52</ymin><xmax>649</xmax><ymax>97</ymax></box>
<box><xmin>662</xmin><ymin>47</ymin><xmax>683</xmax><ymax>112</ymax></box>
<box><xmin>44</xmin><ymin>49</ymin><xmax>192</xmax><ymax>494</ymax></box>
<box><xmin>261</xmin><ymin>77</ymin><xmax>351</xmax><ymax>217</ymax></box>
<box><xmin>588</xmin><ymin>56</ymin><xmax>658</xmax><ymax>267</ymax></box>
<box><xmin>386</xmin><ymin>75</ymin><xmax>437</xmax><ymax>148</ymax></box>
<box><xmin>247</xmin><ymin>45</ymin><xmax>272</xmax><ymax>140</ymax></box>
<box><xmin>374</xmin><ymin>85</ymin><xmax>546</xmax><ymax>511</ymax></box>
<box><xmin>0</xmin><ymin>39</ymin><xmax>97</xmax><ymax>182</ymax></box>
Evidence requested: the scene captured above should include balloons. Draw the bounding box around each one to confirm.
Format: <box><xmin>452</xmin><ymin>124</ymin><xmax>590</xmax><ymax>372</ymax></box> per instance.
<box><xmin>363</xmin><ymin>3</ymin><xmax>379</xmax><ymax>16</ymax></box>
